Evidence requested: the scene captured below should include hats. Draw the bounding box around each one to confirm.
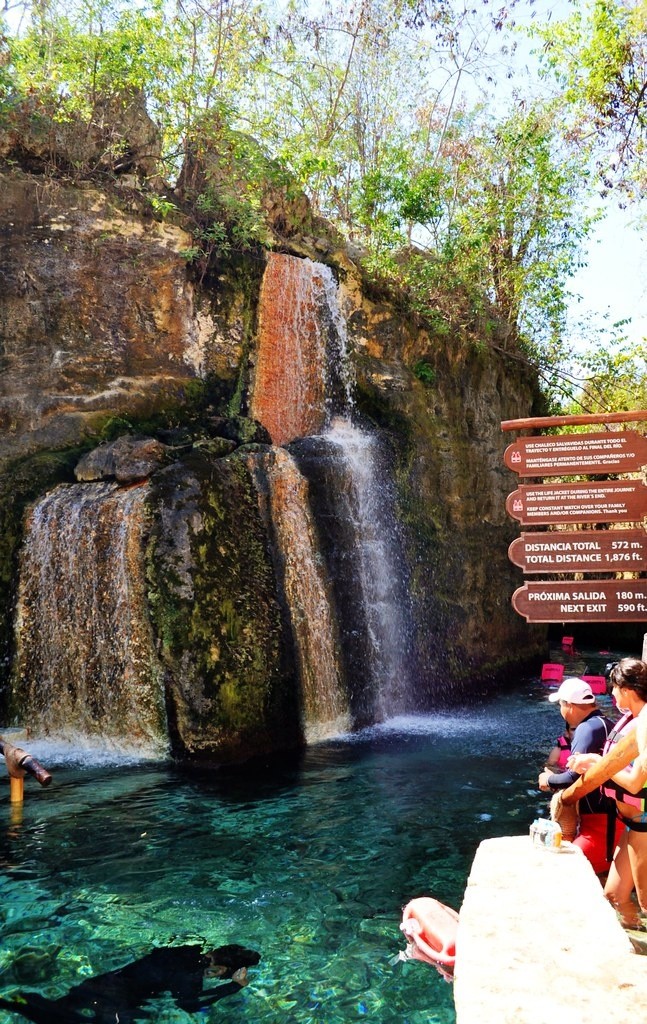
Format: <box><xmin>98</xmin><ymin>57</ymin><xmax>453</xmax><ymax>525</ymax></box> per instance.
<box><xmin>549</xmin><ymin>679</ymin><xmax>595</xmax><ymax>704</ymax></box>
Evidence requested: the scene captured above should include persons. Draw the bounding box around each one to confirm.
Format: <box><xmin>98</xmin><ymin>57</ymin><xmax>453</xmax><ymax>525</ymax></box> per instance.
<box><xmin>538</xmin><ymin>657</ymin><xmax>647</xmax><ymax>928</ymax></box>
<box><xmin>0</xmin><ymin>944</ymin><xmax>261</xmax><ymax>1024</ymax></box>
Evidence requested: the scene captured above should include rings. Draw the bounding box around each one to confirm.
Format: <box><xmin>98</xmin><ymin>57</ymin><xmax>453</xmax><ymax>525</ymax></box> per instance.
<box><xmin>581</xmin><ymin>768</ymin><xmax>587</xmax><ymax>771</ymax></box>
